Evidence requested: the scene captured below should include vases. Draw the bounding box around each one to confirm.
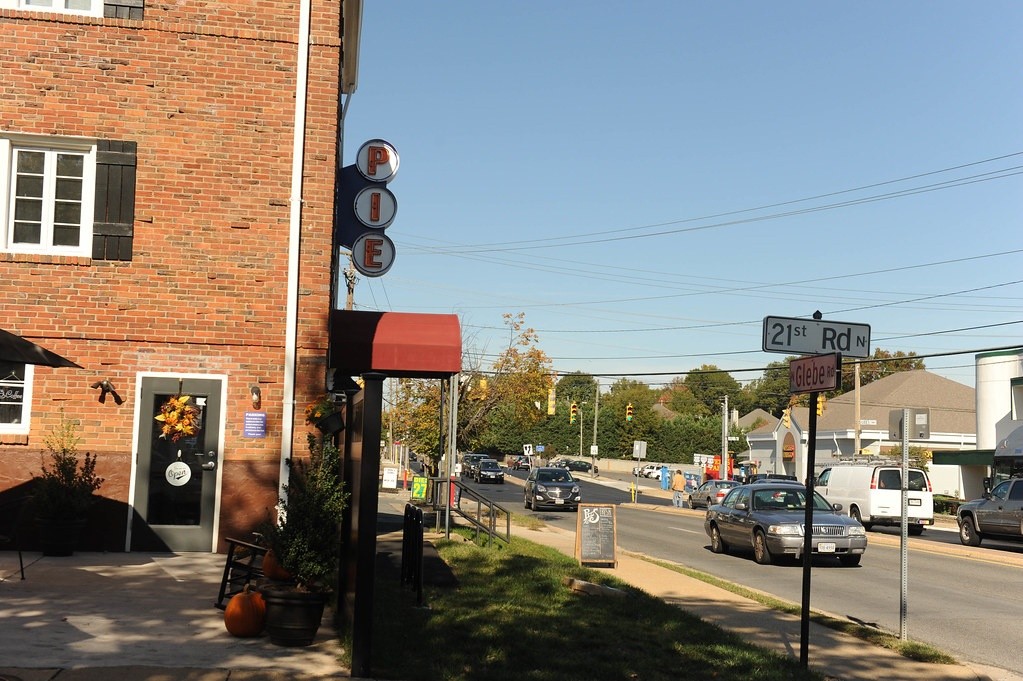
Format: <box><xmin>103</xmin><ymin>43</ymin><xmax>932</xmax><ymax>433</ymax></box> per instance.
<box><xmin>315</xmin><ymin>410</ymin><xmax>345</xmax><ymax>436</ymax></box>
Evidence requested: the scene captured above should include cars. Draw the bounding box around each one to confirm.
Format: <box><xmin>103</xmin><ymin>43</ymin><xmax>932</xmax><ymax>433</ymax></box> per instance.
<box><xmin>752</xmin><ymin>479</ymin><xmax>805</xmax><ymax>486</ymax></box>
<box><xmin>955</xmin><ymin>478</ymin><xmax>1023</xmax><ymax>546</ymax></box>
<box><xmin>704</xmin><ymin>483</ymin><xmax>867</xmax><ymax>568</ymax></box>
<box><xmin>473</xmin><ymin>459</ymin><xmax>504</xmax><ymax>484</ymax></box>
<box><xmin>564</xmin><ymin>461</ymin><xmax>599</xmax><ymax>473</ymax></box>
<box><xmin>511</xmin><ymin>456</ymin><xmax>532</xmax><ymax>471</ymax></box>
<box><xmin>687</xmin><ymin>480</ymin><xmax>742</xmax><ymax>509</ymax></box>
<box><xmin>632</xmin><ymin>465</ymin><xmax>670</xmax><ymax>481</ymax></box>
<box><xmin>461</xmin><ymin>455</ymin><xmax>491</xmax><ymax>478</ymax></box>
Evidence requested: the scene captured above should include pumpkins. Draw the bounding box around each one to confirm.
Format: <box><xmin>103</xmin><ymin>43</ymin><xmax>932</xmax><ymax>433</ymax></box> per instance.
<box><xmin>224</xmin><ymin>582</ymin><xmax>267</xmax><ymax>637</ymax></box>
<box><xmin>262</xmin><ymin>550</ymin><xmax>291</xmax><ymax>580</ymax></box>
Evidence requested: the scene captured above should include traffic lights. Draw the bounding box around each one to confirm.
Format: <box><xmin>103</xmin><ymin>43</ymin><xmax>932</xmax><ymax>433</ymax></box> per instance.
<box><xmin>625</xmin><ymin>406</ymin><xmax>633</xmax><ymax>422</ymax></box>
<box><xmin>570</xmin><ymin>404</ymin><xmax>577</xmax><ymax>424</ymax></box>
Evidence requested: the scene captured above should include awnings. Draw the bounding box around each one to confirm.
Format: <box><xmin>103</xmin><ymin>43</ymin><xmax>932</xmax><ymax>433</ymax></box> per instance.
<box><xmin>994</xmin><ymin>425</ymin><xmax>1023</xmax><ymax>456</ymax></box>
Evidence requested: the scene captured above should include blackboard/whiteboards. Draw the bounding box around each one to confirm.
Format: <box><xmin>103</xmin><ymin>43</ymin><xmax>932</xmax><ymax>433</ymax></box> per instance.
<box><xmin>575</xmin><ymin>503</ymin><xmax>617</xmax><ymax>562</ymax></box>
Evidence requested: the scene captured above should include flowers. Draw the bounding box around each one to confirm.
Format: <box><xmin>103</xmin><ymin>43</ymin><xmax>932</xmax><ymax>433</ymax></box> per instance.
<box><xmin>304</xmin><ymin>394</ymin><xmax>338</xmax><ymax>426</ymax></box>
<box><xmin>154</xmin><ymin>395</ymin><xmax>202</xmax><ymax>443</ymax></box>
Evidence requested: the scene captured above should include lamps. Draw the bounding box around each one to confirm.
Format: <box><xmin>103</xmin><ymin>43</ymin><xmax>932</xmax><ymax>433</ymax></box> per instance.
<box><xmin>251</xmin><ymin>386</ymin><xmax>261</xmax><ymax>403</ymax></box>
<box><xmin>91</xmin><ymin>381</ymin><xmax>116</xmax><ymax>392</ymax></box>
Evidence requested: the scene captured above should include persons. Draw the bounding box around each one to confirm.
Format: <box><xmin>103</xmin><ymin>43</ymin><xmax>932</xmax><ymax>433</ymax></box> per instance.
<box><xmin>672</xmin><ymin>470</ymin><xmax>686</xmax><ymax>508</ymax></box>
<box><xmin>506</xmin><ymin>456</ymin><xmax>513</xmax><ymax>477</ymax></box>
<box><xmin>423</xmin><ymin>459</ymin><xmax>438</xmax><ymax>502</ymax></box>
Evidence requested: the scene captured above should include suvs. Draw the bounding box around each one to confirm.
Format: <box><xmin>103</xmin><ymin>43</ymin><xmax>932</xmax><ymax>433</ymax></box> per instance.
<box><xmin>523</xmin><ymin>466</ymin><xmax>583</xmax><ymax>512</ymax></box>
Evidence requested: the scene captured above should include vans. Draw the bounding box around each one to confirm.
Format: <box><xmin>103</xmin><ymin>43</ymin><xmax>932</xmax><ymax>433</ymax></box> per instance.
<box><xmin>812</xmin><ymin>465</ymin><xmax>935</xmax><ymax>535</ymax></box>
<box><xmin>743</xmin><ymin>474</ymin><xmax>798</xmax><ymax>484</ymax></box>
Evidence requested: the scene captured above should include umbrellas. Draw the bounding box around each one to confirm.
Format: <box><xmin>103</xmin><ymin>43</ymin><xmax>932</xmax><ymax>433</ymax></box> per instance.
<box><xmin>0</xmin><ymin>327</ymin><xmax>84</xmax><ymax>369</ymax></box>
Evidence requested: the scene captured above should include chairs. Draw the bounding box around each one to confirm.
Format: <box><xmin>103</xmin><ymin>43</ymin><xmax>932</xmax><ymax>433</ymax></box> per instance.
<box><xmin>213</xmin><ymin>531</ymin><xmax>270</xmax><ymax>612</ymax></box>
<box><xmin>747</xmin><ymin>496</ymin><xmax>764</xmax><ymax>506</ymax></box>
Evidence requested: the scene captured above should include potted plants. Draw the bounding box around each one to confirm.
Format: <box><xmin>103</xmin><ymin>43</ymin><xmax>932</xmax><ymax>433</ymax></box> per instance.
<box><xmin>260</xmin><ymin>429</ymin><xmax>352</xmax><ymax>648</ymax></box>
<box><xmin>29</xmin><ymin>407</ymin><xmax>105</xmax><ymax>558</ymax></box>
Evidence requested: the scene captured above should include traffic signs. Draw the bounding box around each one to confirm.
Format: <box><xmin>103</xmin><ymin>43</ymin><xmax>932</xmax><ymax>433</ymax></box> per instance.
<box><xmin>788</xmin><ymin>351</ymin><xmax>841</xmax><ymax>396</ymax></box>
<box><xmin>762</xmin><ymin>315</ymin><xmax>872</xmax><ymax>358</ymax></box>
